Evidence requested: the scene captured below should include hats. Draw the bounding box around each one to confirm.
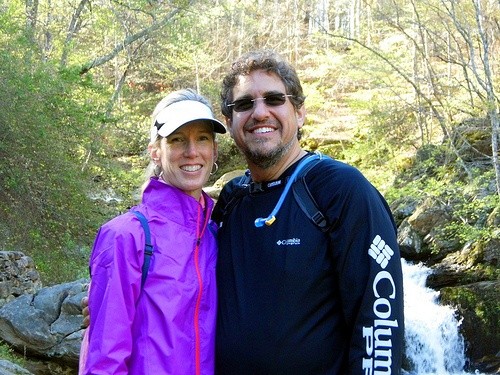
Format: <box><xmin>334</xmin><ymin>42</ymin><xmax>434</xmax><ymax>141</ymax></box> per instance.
<box><xmin>153</xmin><ymin>100</ymin><xmax>227</xmax><ymax>138</ymax></box>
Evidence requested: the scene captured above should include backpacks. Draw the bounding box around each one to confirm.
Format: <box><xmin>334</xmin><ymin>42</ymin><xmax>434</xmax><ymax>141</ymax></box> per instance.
<box><xmin>78</xmin><ymin>211</ymin><xmax>153</xmax><ymax>375</ymax></box>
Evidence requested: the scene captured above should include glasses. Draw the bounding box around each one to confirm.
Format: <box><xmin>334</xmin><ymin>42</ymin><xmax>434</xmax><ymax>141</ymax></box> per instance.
<box><xmin>227</xmin><ymin>93</ymin><xmax>295</xmax><ymax>112</ymax></box>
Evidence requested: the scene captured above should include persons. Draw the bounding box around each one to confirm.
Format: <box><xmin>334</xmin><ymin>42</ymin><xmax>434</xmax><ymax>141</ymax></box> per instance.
<box><xmin>86</xmin><ymin>89</ymin><xmax>216</xmax><ymax>374</ymax></box>
<box><xmin>209</xmin><ymin>57</ymin><xmax>404</xmax><ymax>374</ymax></box>
